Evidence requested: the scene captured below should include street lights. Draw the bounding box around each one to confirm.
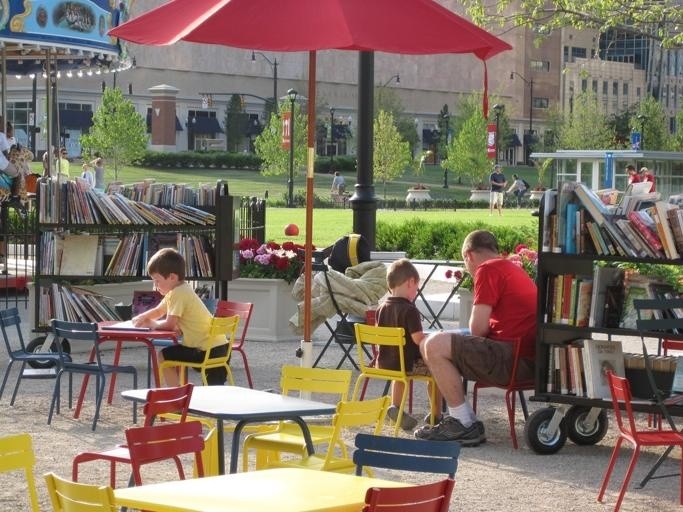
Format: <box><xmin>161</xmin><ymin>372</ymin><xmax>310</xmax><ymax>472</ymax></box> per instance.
<box><xmin>328</xmin><ymin>106</ymin><xmax>335</xmax><ymax>164</ymax></box>
<box><xmin>250</xmin><ymin>50</ymin><xmax>278</xmax><ymax>115</ymax></box>
<box><xmin>510</xmin><ymin>71</ymin><xmax>534</xmax><ymax>167</ymax></box>
<box><xmin>493</xmin><ymin>103</ymin><xmax>505</xmax><ymax>166</ymax></box>
<box><xmin>377</xmin><ymin>73</ymin><xmax>401</xmax><ymax>114</ymax></box>
<box><xmin>441</xmin><ymin>114</ymin><xmax>449</xmax><ymax>189</ymax></box>
<box><xmin>637</xmin><ymin>115</ymin><xmax>648</xmax><ymax>168</ymax></box>
<box><xmin>286</xmin><ymin>88</ymin><xmax>299</xmax><ymax>208</ymax></box>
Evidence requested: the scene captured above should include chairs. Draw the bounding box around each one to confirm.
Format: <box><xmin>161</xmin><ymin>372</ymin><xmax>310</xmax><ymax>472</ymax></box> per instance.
<box><xmin>72</xmin><ymin>382</ymin><xmax>193</xmax><ymax>488</ymax></box>
<box><xmin>598</xmin><ymin>371</ymin><xmax>683</xmax><ymax>512</ymax></box>
<box><xmin>264</xmin><ymin>394</ymin><xmax>390</xmax><ymax>479</ymax></box>
<box><xmin>299</xmin><ymin>246</ymin><xmax>535</xmax><ymax>449</ymax></box>
<box><xmin>242</xmin><ymin>364</ymin><xmax>352</xmax><ymax>472</ymax></box>
<box><xmin>126</xmin><ymin>420</ymin><xmax>206</xmax><ymax>486</ymax></box>
<box><xmin>44</xmin><ymin>471</ymin><xmax>118</xmax><ymax>511</ymax></box>
<box><xmin>353</xmin><ymin>432</ymin><xmax>461</xmax><ymax>482</ymax></box>
<box><xmin>1</xmin><ymin>432</ymin><xmax>42</xmax><ymax>511</ymax></box>
<box><xmin>362</xmin><ymin>477</ymin><xmax>456</xmax><ymax>512</ymax></box>
<box><xmin>2</xmin><ymin>290</ymin><xmax>253</xmax><ymax>431</ymax></box>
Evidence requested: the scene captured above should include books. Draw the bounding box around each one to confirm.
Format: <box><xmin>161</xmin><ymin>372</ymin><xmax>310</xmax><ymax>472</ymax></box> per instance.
<box><xmin>38</xmin><ymin>178</ymin><xmax>224</xmax><ymax>327</ymax></box>
<box><xmin>541</xmin><ymin>180</ymin><xmax>683</xmax><ymax>406</ymax></box>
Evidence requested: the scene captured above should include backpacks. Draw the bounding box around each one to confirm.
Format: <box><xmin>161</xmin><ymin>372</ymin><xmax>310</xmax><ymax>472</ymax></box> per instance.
<box><xmin>321</xmin><ymin>233</ymin><xmax>370</xmax><ymax>274</ymax></box>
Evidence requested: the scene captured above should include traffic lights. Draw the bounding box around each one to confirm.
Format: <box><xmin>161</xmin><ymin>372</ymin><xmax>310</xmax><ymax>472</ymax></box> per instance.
<box><xmin>208</xmin><ymin>98</ymin><xmax>211</xmax><ymax>107</ymax></box>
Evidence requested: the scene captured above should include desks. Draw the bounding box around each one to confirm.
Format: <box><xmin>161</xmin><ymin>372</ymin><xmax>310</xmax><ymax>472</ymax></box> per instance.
<box><xmin>120</xmin><ymin>384</ymin><xmax>338</xmax><ymax>476</ymax></box>
<box><xmin>114</xmin><ymin>467</ymin><xmax>418</xmax><ymax>510</ymax></box>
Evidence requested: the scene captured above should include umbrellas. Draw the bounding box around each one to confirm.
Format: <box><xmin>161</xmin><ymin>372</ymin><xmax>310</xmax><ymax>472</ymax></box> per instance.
<box><xmin>106</xmin><ymin>0</ymin><xmax>514</xmax><ymax>401</ymax></box>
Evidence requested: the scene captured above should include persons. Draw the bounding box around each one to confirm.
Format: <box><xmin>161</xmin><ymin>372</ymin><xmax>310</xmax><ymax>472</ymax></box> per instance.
<box><xmin>626</xmin><ymin>165</ymin><xmax>641</xmax><ymax>184</ymax></box>
<box><xmin>506</xmin><ymin>174</ymin><xmax>527</xmax><ymax>209</ymax></box>
<box><xmin>0</xmin><ymin>115</ymin><xmax>104</xmax><ymax>275</ymax></box>
<box><xmin>331</xmin><ymin>171</ymin><xmax>346</xmax><ymax>201</ymax></box>
<box><xmin>489</xmin><ymin>165</ymin><xmax>507</xmax><ymax>217</ymax></box>
<box><xmin>415</xmin><ymin>229</ymin><xmax>538</xmax><ymax>447</ymax></box>
<box><xmin>640</xmin><ymin>167</ymin><xmax>655</xmax><ymax>193</ymax></box>
<box><xmin>132</xmin><ymin>247</ymin><xmax>229</xmax><ymax>388</ymax></box>
<box><xmin>375</xmin><ymin>259</ymin><xmax>444</xmax><ymax>431</ymax></box>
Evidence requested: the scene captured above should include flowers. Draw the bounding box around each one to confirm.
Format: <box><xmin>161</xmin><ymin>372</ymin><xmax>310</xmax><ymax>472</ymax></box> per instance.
<box><xmin>235</xmin><ymin>235</ymin><xmax>314</xmax><ymax>280</ymax></box>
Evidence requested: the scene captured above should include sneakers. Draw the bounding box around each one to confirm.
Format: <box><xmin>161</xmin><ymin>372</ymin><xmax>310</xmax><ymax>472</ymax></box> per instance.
<box><xmin>11</xmin><ymin>170</ymin><xmax>24</xmax><ymax>198</ymax></box>
<box><xmin>414</xmin><ymin>412</ymin><xmax>487</xmax><ymax>447</ymax></box>
<box><xmin>386</xmin><ymin>403</ymin><xmax>418</xmax><ymax>431</ymax></box>
